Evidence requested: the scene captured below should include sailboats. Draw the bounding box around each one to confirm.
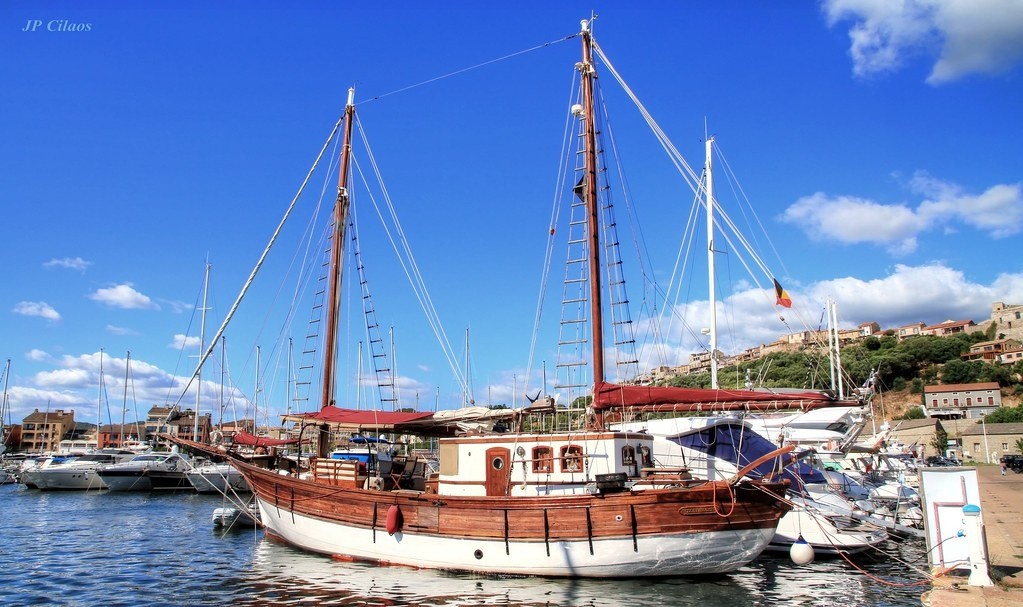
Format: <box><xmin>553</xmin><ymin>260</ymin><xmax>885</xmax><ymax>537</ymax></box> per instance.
<box><xmin>0</xmin><ymin>8</ymin><xmax>937</xmax><ymax>581</ymax></box>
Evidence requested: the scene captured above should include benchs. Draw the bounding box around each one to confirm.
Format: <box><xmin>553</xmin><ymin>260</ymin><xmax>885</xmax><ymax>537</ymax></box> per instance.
<box><xmin>312</xmin><ymin>457</ymin><xmax>367</xmax><ymax>489</ymax></box>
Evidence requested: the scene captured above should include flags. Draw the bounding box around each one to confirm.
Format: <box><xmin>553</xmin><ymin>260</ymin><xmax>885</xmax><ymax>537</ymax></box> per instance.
<box><xmin>908</xmin><ymin>442</ymin><xmax>918</xmax><ymax>458</ymax></box>
<box><xmin>773</xmin><ymin>278</ymin><xmax>792</xmax><ymax>308</ymax></box>
<box><xmin>866</xmin><ymin>456</ymin><xmax>873</xmax><ymax>473</ymax></box>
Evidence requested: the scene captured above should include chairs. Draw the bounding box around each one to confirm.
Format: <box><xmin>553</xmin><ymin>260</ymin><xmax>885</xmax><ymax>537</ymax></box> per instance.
<box><xmin>390</xmin><ymin>456</ymin><xmax>418</xmax><ymax>490</ymax></box>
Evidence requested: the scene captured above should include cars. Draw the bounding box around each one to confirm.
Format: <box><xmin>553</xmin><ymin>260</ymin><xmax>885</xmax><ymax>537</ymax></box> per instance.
<box><xmin>925</xmin><ymin>455</ymin><xmax>963</xmax><ymax>467</ymax></box>
<box><xmin>1000</xmin><ymin>455</ymin><xmax>1023</xmax><ymax>474</ymax></box>
